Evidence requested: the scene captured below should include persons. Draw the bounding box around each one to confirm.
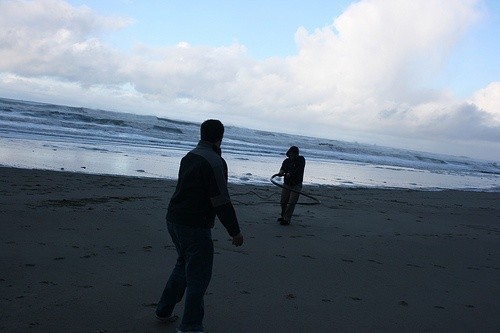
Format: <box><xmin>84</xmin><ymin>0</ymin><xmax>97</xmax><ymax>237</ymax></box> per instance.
<box><xmin>277</xmin><ymin>146</ymin><xmax>306</xmax><ymax>227</ymax></box>
<box><xmin>153</xmin><ymin>118</ymin><xmax>245</xmax><ymax>333</ymax></box>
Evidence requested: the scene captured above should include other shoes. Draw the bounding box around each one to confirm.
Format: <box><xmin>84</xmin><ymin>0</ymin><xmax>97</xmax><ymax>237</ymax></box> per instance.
<box><xmin>278</xmin><ymin>218</ymin><xmax>290</xmax><ymax>225</ymax></box>
<box><xmin>157</xmin><ymin>314</ymin><xmax>179</xmax><ymax>322</ymax></box>
